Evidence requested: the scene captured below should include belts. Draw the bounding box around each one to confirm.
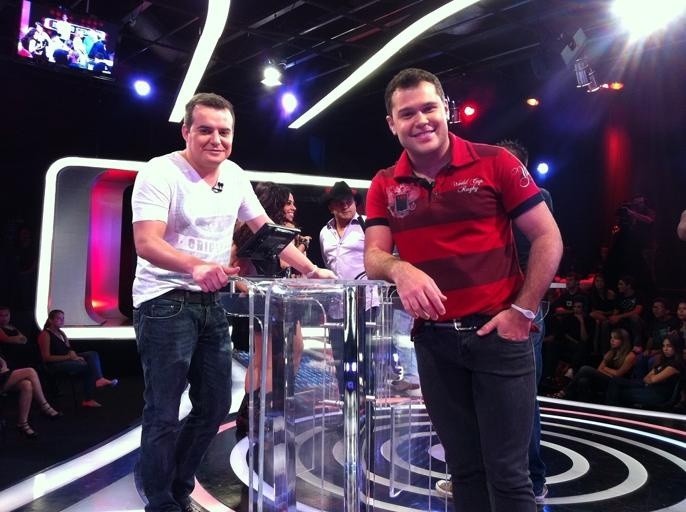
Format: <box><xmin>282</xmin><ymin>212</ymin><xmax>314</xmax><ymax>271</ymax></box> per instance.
<box><xmin>424</xmin><ymin>316</ymin><xmax>490</xmax><ymax>332</ymax></box>
<box><xmin>159</xmin><ymin>288</ymin><xmax>221</xmax><ymax>304</ymax></box>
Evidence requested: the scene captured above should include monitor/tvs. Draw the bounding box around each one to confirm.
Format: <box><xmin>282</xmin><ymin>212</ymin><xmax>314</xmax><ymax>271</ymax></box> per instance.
<box><xmin>236</xmin><ymin>223</ymin><xmax>301</xmax><ymax>260</ymax></box>
<box><xmin>15</xmin><ymin>0</ymin><xmax>129</xmax><ymax>83</ymax></box>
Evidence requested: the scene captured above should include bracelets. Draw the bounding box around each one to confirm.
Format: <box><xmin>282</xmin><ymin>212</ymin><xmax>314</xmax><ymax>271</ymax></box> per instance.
<box><xmin>307</xmin><ymin>265</ymin><xmax>319</xmax><ymax>277</ymax></box>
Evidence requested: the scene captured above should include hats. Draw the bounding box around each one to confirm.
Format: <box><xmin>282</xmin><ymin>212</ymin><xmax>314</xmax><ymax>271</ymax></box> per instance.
<box><xmin>321</xmin><ymin>181</ymin><xmax>362</xmax><ymax>207</ymax></box>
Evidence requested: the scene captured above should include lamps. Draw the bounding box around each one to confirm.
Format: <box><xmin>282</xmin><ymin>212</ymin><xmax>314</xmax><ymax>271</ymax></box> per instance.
<box><xmin>571</xmin><ymin>55</ymin><xmax>626</xmax><ymax>94</ymax></box>
<box><xmin>446</xmin><ymin>96</ymin><xmax>462</xmax><ymax>126</ymax></box>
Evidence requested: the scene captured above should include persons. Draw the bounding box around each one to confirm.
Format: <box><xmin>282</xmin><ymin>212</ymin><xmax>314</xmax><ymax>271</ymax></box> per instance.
<box><xmin>130</xmin><ymin>92</ymin><xmax>341</xmax><ymax>512</ymax></box>
<box><xmin>0</xmin><ymin>357</ymin><xmax>63</xmax><ymax>436</ymax></box>
<box><xmin>225</xmin><ymin>180</ymin><xmax>304</xmax><ymax>411</ymax></box>
<box><xmin>362</xmin><ymin>67</ymin><xmax>563</xmax><ymax>512</ymax></box>
<box><xmin>0</xmin><ymin>307</ymin><xmax>37</xmax><ymax>368</ymax></box>
<box><xmin>233</xmin><ymin>184</ymin><xmax>314</xmax><ymax>435</ymax></box>
<box><xmin>21</xmin><ymin>13</ymin><xmax>114</xmax><ymax>75</ymax></box>
<box><xmin>37</xmin><ymin>308</ymin><xmax>118</xmax><ymax>407</ymax></box>
<box><xmin>434</xmin><ymin>140</ymin><xmax>554</xmax><ymax>505</ymax></box>
<box><xmin>537</xmin><ymin>190</ymin><xmax>686</xmax><ymax>403</ymax></box>
<box><xmin>319</xmin><ymin>179</ymin><xmax>389</xmax><ymax>441</ymax></box>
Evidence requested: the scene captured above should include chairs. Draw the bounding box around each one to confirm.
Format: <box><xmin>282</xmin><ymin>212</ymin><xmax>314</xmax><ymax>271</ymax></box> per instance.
<box><xmin>29</xmin><ymin>331</ymin><xmax>83</xmax><ymax>409</ymax></box>
<box><xmin>637</xmin><ymin>379</ymin><xmax>682</xmax><ymax>408</ymax></box>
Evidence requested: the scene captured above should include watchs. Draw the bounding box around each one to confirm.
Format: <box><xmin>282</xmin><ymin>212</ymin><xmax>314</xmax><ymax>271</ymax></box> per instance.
<box><xmin>511</xmin><ymin>304</ymin><xmax>538</xmax><ymax>320</ymax></box>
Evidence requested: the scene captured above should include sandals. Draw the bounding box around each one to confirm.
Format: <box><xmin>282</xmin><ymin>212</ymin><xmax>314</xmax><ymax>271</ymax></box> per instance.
<box><xmin>544</xmin><ymin>392</ymin><xmax>565</xmax><ymax>399</ymax></box>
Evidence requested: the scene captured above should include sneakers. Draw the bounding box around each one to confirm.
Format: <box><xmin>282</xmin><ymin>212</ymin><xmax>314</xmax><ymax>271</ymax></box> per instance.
<box><xmin>95</xmin><ymin>379</ymin><xmax>118</xmax><ymax>391</ymax></box>
<box><xmin>535</xmin><ymin>484</ymin><xmax>548</xmax><ymax>504</ymax></box>
<box><xmin>183</xmin><ymin>504</ymin><xmax>203</xmax><ymax>512</ymax></box>
<box><xmin>436</xmin><ymin>480</ymin><xmax>453</xmax><ymax>498</ymax></box>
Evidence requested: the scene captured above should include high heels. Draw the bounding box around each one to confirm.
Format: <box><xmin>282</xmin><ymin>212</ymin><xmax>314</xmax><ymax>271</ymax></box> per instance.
<box><xmin>39</xmin><ymin>402</ymin><xmax>63</xmax><ymax>419</ymax></box>
<box><xmin>16</xmin><ymin>421</ymin><xmax>40</xmax><ymax>438</ymax></box>
